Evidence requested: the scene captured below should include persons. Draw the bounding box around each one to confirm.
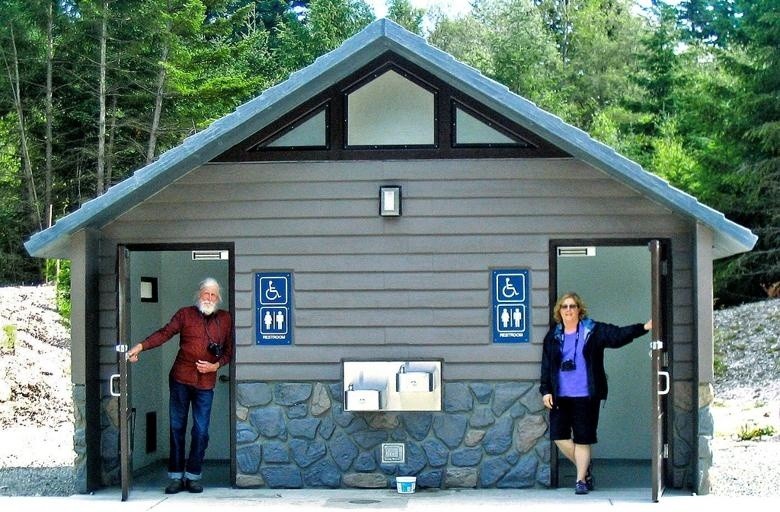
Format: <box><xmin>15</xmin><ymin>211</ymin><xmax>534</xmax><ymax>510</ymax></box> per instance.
<box><xmin>539</xmin><ymin>290</ymin><xmax>653</xmax><ymax>496</ymax></box>
<box><xmin>124</xmin><ymin>276</ymin><xmax>234</xmax><ymax>495</ymax></box>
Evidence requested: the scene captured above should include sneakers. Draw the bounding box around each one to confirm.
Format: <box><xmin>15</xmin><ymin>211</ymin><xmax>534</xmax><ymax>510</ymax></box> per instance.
<box><xmin>185</xmin><ymin>479</ymin><xmax>204</xmax><ymax>493</ymax></box>
<box><xmin>585</xmin><ymin>459</ymin><xmax>595</xmax><ymax>490</ymax></box>
<box><xmin>575</xmin><ymin>479</ymin><xmax>588</xmax><ymax>494</ymax></box>
<box><xmin>165</xmin><ymin>480</ymin><xmax>184</xmax><ymax>494</ymax></box>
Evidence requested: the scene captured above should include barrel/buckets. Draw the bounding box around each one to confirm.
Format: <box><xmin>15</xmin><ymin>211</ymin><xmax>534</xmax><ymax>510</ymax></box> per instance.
<box><xmin>395</xmin><ymin>476</ymin><xmax>417</xmax><ymax>494</ymax></box>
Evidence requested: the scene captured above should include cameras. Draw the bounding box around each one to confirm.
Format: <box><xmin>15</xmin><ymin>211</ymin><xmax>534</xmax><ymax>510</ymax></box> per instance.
<box><xmin>208</xmin><ymin>342</ymin><xmax>226</xmax><ymax>359</ymax></box>
<box><xmin>561</xmin><ymin>358</ymin><xmax>576</xmax><ymax>371</ymax></box>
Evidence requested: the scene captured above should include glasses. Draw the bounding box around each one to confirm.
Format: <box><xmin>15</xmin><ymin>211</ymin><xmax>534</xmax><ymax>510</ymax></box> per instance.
<box><xmin>560</xmin><ymin>304</ymin><xmax>578</xmax><ymax>309</ymax></box>
